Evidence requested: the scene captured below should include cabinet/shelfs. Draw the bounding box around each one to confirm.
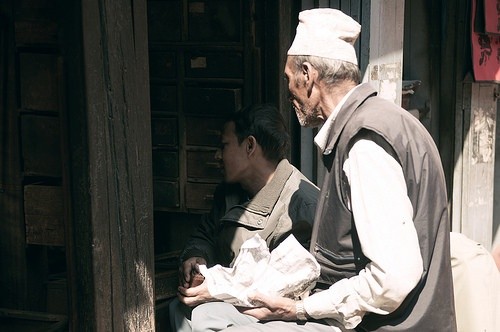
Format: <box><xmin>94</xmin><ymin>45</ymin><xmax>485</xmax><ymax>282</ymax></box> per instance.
<box><xmin>0</xmin><ymin>0</ymin><xmax>273</xmax><ymax>332</ymax></box>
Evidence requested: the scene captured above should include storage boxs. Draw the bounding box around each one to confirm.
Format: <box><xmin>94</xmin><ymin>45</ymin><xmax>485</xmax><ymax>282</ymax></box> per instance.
<box><xmin>23</xmin><ymin>182</ymin><xmax>67</xmax><ymax>247</ymax></box>
<box><xmin>156</xmin><ymin>271</ymin><xmax>180</xmax><ymax>301</ymax></box>
<box><xmin>13</xmin><ymin>0</ymin><xmax>63</xmax><ymax>44</ymax></box>
<box><xmin>45</xmin><ymin>280</ymin><xmax>69</xmax><ymax>315</ymax></box>
<box><xmin>20</xmin><ymin>115</ymin><xmax>63</xmax><ymax>175</ymax></box>
<box><xmin>19</xmin><ymin>53</ymin><xmax>61</xmax><ymax>112</ymax></box>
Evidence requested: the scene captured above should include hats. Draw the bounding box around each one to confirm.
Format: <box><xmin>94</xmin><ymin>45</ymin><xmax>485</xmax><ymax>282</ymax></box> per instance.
<box><xmin>287</xmin><ymin>7</ymin><xmax>362</xmax><ymax>66</ymax></box>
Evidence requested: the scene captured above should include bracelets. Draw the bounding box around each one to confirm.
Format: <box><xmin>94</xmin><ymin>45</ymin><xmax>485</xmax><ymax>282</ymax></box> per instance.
<box><xmin>295</xmin><ymin>300</ymin><xmax>308</xmax><ymax>320</ymax></box>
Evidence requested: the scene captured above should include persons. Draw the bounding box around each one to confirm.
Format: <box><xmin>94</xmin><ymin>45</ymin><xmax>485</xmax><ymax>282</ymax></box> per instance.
<box><xmin>191</xmin><ymin>8</ymin><xmax>458</xmax><ymax>332</ymax></box>
<box><xmin>168</xmin><ymin>103</ymin><xmax>321</xmax><ymax>332</ymax></box>
